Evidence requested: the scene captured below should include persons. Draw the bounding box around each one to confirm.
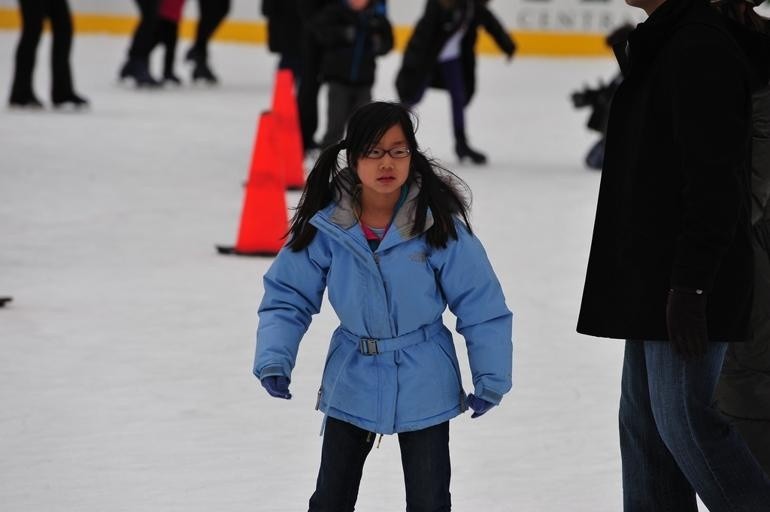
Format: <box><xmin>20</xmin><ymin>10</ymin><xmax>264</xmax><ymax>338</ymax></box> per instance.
<box><xmin>396</xmin><ymin>0</ymin><xmax>517</xmax><ymax>164</ymax></box>
<box><xmin>121</xmin><ymin>1</ymin><xmax>185</xmax><ymax>87</ymax></box>
<box><xmin>257</xmin><ymin>104</ymin><xmax>511</xmax><ymax>512</ymax></box>
<box><xmin>576</xmin><ymin>1</ymin><xmax>769</xmax><ymax>512</ymax></box>
<box><xmin>9</xmin><ymin>0</ymin><xmax>85</xmax><ymax>107</ymax></box>
<box><xmin>715</xmin><ymin>0</ymin><xmax>770</xmax><ymax>468</ymax></box>
<box><xmin>187</xmin><ymin>1</ymin><xmax>230</xmax><ymax>82</ymax></box>
<box><xmin>260</xmin><ymin>1</ymin><xmax>392</xmax><ymax>150</ymax></box>
<box><xmin>572</xmin><ymin>73</ymin><xmax>625</xmax><ymax>169</ymax></box>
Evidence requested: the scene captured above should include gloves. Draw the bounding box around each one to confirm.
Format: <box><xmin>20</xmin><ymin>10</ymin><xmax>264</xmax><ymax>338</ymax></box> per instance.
<box><xmin>467</xmin><ymin>393</ymin><xmax>494</xmax><ymax>418</ymax></box>
<box><xmin>261</xmin><ymin>376</ymin><xmax>293</xmax><ymax>400</ymax></box>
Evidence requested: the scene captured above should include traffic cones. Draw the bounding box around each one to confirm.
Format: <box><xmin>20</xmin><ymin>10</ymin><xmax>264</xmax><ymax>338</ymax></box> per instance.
<box><xmin>215</xmin><ymin>110</ymin><xmax>290</xmax><ymax>258</ymax></box>
<box><xmin>243</xmin><ymin>58</ymin><xmax>308</xmax><ymax>191</ymax></box>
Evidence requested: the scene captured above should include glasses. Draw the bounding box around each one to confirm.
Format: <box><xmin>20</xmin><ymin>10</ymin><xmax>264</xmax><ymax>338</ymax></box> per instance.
<box><xmin>359</xmin><ymin>145</ymin><xmax>412</xmax><ymax>158</ymax></box>
